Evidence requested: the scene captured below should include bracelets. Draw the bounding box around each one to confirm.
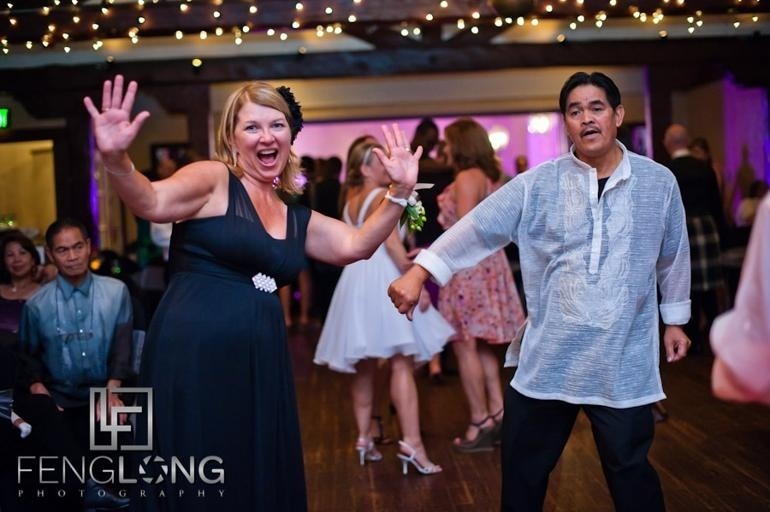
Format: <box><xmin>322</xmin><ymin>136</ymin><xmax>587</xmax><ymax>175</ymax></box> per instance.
<box><xmin>386</xmin><ymin>194</ymin><xmax>408</xmax><ymax>207</ymax></box>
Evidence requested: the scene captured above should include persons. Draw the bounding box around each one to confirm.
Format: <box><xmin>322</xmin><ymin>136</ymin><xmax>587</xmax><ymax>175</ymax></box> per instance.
<box><xmin>84</xmin><ymin>74</ymin><xmax>423</xmax><ymax>512</ymax></box>
<box><xmin>386</xmin><ymin>70</ymin><xmax>693</xmax><ymax>512</ymax></box>
<box><xmin>658</xmin><ymin>123</ymin><xmax>767</xmax><ymax>352</ymax></box>
<box><xmin>710</xmin><ymin>190</ymin><xmax>769</xmax><ymax>406</ymax></box>
<box><xmin>292</xmin><ymin>111</ymin><xmax>529</xmax><ymax>478</ymax></box>
<box><xmin>0</xmin><ymin>140</ymin><xmax>192</xmax><ymax>512</ymax></box>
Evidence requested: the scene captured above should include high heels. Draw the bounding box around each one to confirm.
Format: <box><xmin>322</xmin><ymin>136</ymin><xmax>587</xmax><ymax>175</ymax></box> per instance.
<box><xmin>449</xmin><ymin>408</ymin><xmax>504</xmax><ymax>460</ymax></box>
<box><xmin>395</xmin><ymin>440</ymin><xmax>443</xmax><ymax>476</ymax></box>
<box><xmin>356</xmin><ymin>435</ymin><xmax>382</xmax><ymax>466</ymax></box>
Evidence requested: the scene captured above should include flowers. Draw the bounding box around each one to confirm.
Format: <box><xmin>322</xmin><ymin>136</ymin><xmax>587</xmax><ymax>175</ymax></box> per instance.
<box><xmin>398</xmin><ymin>196</ymin><xmax>430</xmax><ymax>235</ymax></box>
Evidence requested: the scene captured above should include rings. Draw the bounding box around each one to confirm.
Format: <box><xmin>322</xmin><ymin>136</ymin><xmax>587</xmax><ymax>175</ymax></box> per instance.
<box><xmin>101</xmin><ymin>106</ymin><xmax>111</xmax><ymax>112</ymax></box>
